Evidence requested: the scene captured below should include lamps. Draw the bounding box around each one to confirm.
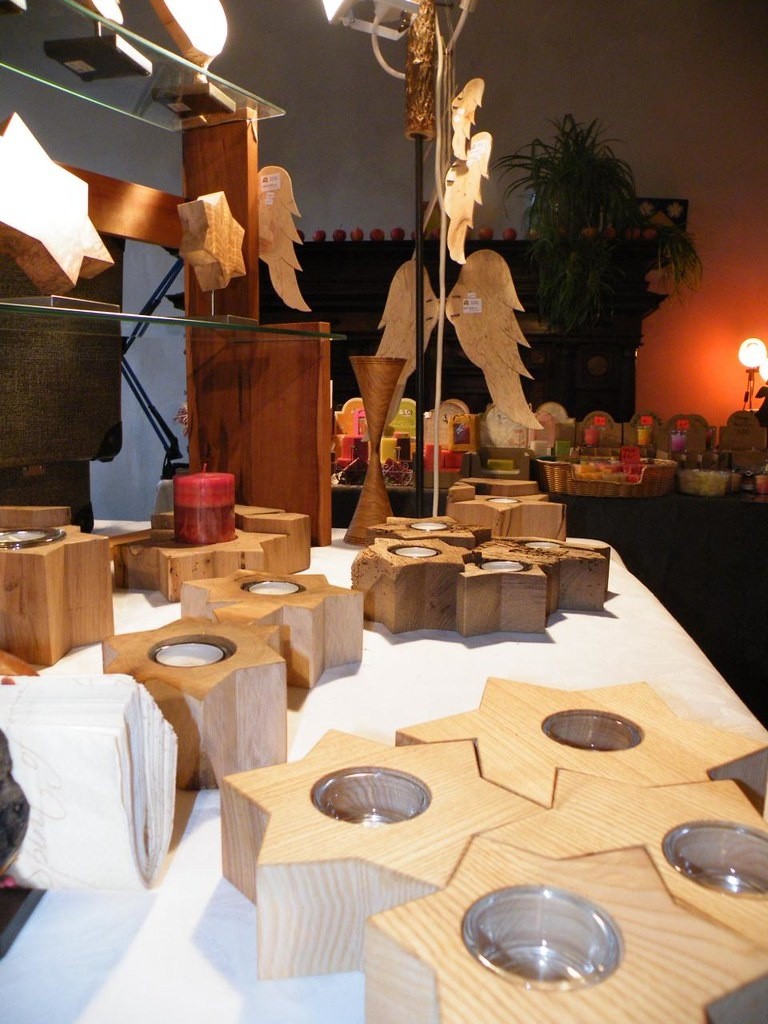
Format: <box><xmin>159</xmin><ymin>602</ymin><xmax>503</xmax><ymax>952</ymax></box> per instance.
<box><xmin>738</xmin><ymin>338</ymin><xmax>768</xmax><ymax>413</ymax></box>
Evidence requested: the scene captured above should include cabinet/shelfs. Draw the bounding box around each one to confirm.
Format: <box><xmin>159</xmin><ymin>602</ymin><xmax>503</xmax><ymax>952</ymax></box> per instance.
<box><xmin>0</xmin><ymin>0</ymin><xmax>346</xmax><ymax>547</ymax></box>
<box><xmin>166</xmin><ymin>238</ymin><xmax>672</xmax><ymax>423</ymax></box>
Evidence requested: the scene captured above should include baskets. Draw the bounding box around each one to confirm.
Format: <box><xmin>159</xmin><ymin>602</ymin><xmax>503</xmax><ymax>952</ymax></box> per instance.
<box><xmin>535</xmin><ymin>456</ymin><xmax>677</xmax><ymax>497</ymax></box>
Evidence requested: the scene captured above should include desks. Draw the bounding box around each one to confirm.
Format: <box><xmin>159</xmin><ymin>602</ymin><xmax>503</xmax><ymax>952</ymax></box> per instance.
<box><xmin>1</xmin><ymin>540</ymin><xmax>768</xmax><ymax>1024</ymax></box>
<box><xmin>330</xmin><ymin>484</ymin><xmax>768</xmax><ymax>736</ymax></box>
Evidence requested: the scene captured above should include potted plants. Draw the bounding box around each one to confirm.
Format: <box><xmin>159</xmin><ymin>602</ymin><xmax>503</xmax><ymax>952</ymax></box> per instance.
<box><xmin>492</xmin><ymin>112</ymin><xmax>704</xmax><ymax>335</ymax></box>
